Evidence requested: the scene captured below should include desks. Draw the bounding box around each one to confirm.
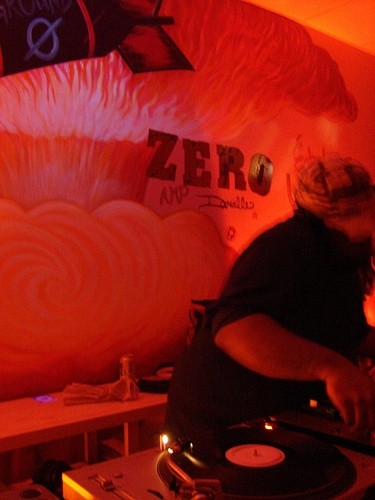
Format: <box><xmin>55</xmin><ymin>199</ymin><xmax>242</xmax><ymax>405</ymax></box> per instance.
<box><xmin>0</xmin><ymin>382</ymin><xmax>169</xmax><ymax>480</ymax></box>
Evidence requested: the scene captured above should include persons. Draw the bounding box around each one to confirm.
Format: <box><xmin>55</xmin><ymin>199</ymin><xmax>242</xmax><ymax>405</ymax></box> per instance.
<box><xmin>166</xmin><ymin>151</ymin><xmax>375</xmax><ymax>437</ymax></box>
